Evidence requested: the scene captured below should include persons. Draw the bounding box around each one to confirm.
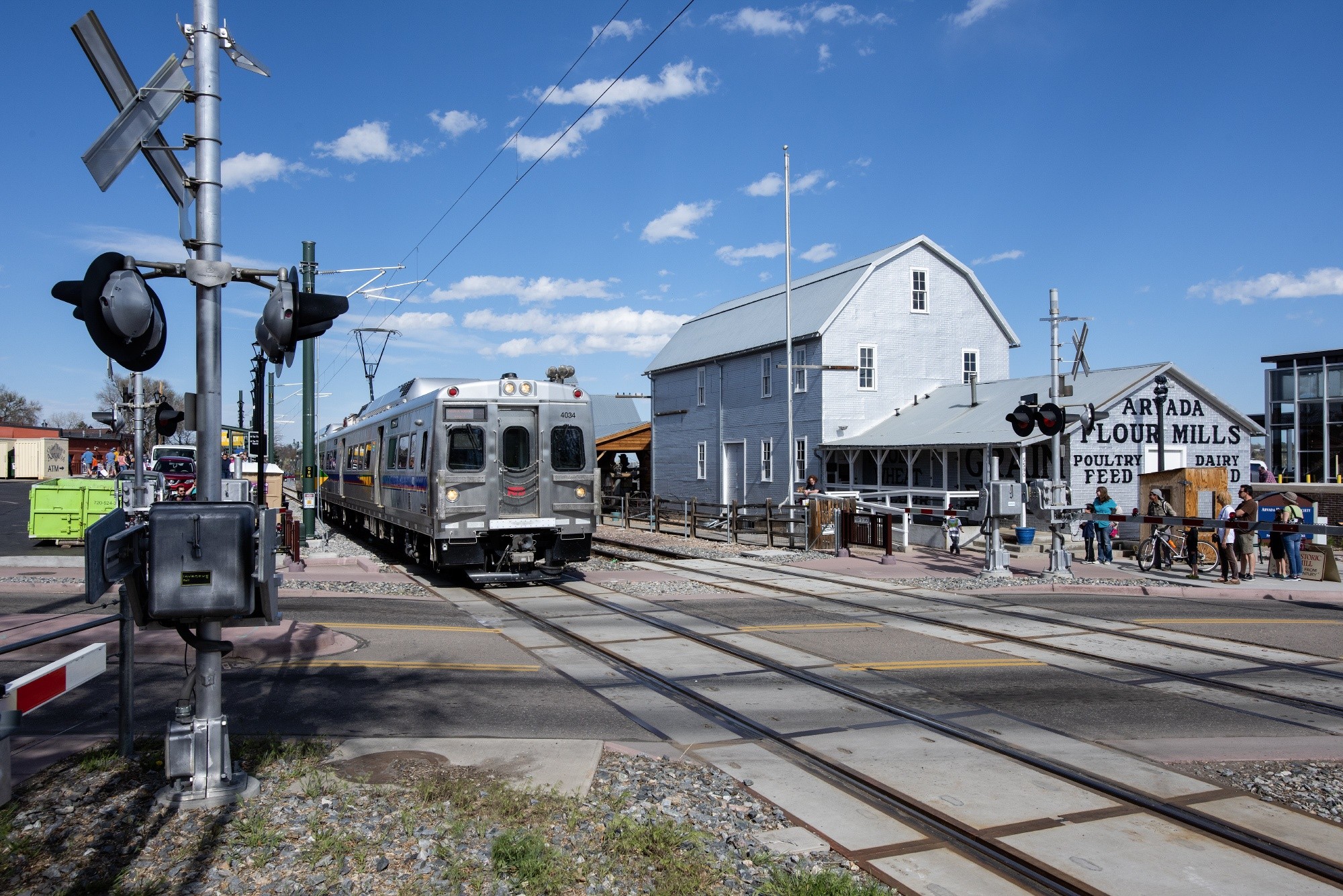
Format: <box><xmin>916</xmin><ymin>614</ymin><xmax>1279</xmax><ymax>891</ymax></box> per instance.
<box><xmin>1211</xmin><ymin>490</ymin><xmax>1241</xmax><ymax>585</ymax></box>
<box><xmin>1258</xmin><ymin>466</ymin><xmax>1277</xmax><ymax>483</ymax></box>
<box><xmin>946</xmin><ymin>516</ymin><xmax>964</xmax><ymax>556</ymax></box>
<box><xmin>1079</xmin><ymin>503</ymin><xmax>1095</xmax><ymax>564</ymax></box>
<box><xmin>802</xmin><ymin>475</ymin><xmax>826</xmax><ymax>519</ymax></box>
<box><xmin>1233</xmin><ymin>484</ymin><xmax>1257</xmax><ymax>581</ymax></box>
<box><xmin>168</xmin><ymin>485</ymin><xmax>191</xmax><ymax>502</ymax></box>
<box><xmin>221</xmin><ymin>451</ymin><xmax>249</xmax><ymax>479</ymax></box>
<box><xmin>1146</xmin><ymin>489</ymin><xmax>1176</xmax><ymax>570</ymax></box>
<box><xmin>1268</xmin><ymin>510</ymin><xmax>1287</xmax><ymax>579</ymax></box>
<box><xmin>1181</xmin><ymin>517</ymin><xmax>1199</xmax><ymax>579</ymax></box>
<box><xmin>1280</xmin><ymin>492</ymin><xmax>1303</xmax><ymax>582</ymax></box>
<box><xmin>69</xmin><ymin>447</ymin><xmax>152</xmax><ymax>479</ymax></box>
<box><xmin>1092</xmin><ymin>486</ymin><xmax>1122</xmax><ymax>565</ymax></box>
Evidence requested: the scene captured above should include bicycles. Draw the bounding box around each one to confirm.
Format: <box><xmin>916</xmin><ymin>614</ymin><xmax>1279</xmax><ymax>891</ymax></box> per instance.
<box><xmin>1137</xmin><ymin>524</ymin><xmax>1219</xmax><ymax>572</ymax></box>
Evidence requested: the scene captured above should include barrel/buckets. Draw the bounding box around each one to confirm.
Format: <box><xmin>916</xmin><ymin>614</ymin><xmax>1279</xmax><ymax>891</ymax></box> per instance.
<box><xmin>1015</xmin><ymin>527</ymin><xmax>1036</xmax><ymax>545</ymax></box>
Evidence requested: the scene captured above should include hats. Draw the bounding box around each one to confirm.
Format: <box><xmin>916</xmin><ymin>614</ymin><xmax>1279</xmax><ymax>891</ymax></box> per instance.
<box><xmin>1150</xmin><ymin>488</ymin><xmax>1164</xmax><ymax>500</ymax></box>
<box><xmin>1280</xmin><ymin>491</ymin><xmax>1299</xmax><ymax>505</ymax></box>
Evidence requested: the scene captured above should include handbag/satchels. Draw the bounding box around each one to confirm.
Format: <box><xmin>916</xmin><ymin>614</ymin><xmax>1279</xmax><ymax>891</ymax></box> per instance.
<box><xmin>1110</xmin><ymin>527</ymin><xmax>1117</xmax><ymax>537</ymax></box>
<box><xmin>1211</xmin><ymin>533</ymin><xmax>1218</xmax><ymax>543</ymax></box>
<box><xmin>1283</xmin><ymin>506</ymin><xmax>1304</xmax><ymax>538</ymax></box>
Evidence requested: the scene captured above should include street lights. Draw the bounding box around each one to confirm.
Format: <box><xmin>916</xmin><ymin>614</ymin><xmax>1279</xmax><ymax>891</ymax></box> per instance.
<box><xmin>1152</xmin><ymin>375</ymin><xmax>1169</xmax><ymax>472</ymax></box>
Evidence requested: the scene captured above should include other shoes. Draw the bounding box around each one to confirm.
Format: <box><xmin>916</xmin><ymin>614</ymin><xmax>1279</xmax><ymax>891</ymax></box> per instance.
<box><xmin>1189</xmin><ymin>575</ymin><xmax>1200</xmax><ymax>579</ymax></box>
<box><xmin>1269</xmin><ymin>573</ymin><xmax>1281</xmax><ymax>579</ymax></box>
<box><xmin>1092</xmin><ymin>559</ymin><xmax>1104</xmax><ymax>564</ymax></box>
<box><xmin>1229</xmin><ymin>572</ymin><xmax>1246</xmax><ymax>579</ymax></box>
<box><xmin>1241</xmin><ymin>573</ymin><xmax>1256</xmax><ymax>581</ymax></box>
<box><xmin>1281</xmin><ymin>574</ymin><xmax>1298</xmax><ymax>582</ymax></box>
<box><xmin>1104</xmin><ymin>559</ymin><xmax>1112</xmax><ymax>565</ymax></box>
<box><xmin>1081</xmin><ymin>560</ymin><xmax>1093</xmax><ymax>564</ymax></box>
<box><xmin>1186</xmin><ymin>573</ymin><xmax>1195</xmax><ymax>578</ymax></box>
<box><xmin>1149</xmin><ymin>563</ymin><xmax>1160</xmax><ymax>569</ymax></box>
<box><xmin>1211</xmin><ymin>576</ymin><xmax>1228</xmax><ymax>583</ymax></box>
<box><xmin>1163</xmin><ymin>564</ymin><xmax>1172</xmax><ymax>571</ymax></box>
<box><xmin>1276</xmin><ymin>574</ymin><xmax>1288</xmax><ymax>579</ymax></box>
<box><xmin>1224</xmin><ymin>579</ymin><xmax>1241</xmax><ymax>585</ymax></box>
<box><xmin>1296</xmin><ymin>575</ymin><xmax>1302</xmax><ymax>581</ymax></box>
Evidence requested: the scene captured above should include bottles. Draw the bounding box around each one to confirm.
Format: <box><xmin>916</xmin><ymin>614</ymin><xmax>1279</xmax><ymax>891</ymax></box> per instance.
<box><xmin>1168</xmin><ymin>539</ymin><xmax>1176</xmax><ymax>548</ymax></box>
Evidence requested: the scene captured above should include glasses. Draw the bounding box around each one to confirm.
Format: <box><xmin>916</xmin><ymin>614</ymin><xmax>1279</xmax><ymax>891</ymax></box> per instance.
<box><xmin>1096</xmin><ymin>490</ymin><xmax>1100</xmax><ymax>492</ymax></box>
<box><xmin>1217</xmin><ymin>495</ymin><xmax>1219</xmax><ymax>499</ymax></box>
<box><xmin>1239</xmin><ymin>490</ymin><xmax>1245</xmax><ymax>493</ymax></box>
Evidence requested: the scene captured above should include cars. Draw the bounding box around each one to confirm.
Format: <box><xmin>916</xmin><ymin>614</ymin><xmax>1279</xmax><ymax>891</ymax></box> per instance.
<box><xmin>113</xmin><ymin>469</ymin><xmax>170</xmax><ymax>504</ymax></box>
<box><xmin>151</xmin><ymin>456</ymin><xmax>197</xmax><ymax>502</ymax></box>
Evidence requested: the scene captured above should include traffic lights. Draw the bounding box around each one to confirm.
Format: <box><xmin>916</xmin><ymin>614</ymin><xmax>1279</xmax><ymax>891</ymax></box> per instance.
<box><xmin>255</xmin><ymin>282</ymin><xmax>349</xmax><ymax>366</ymax></box>
<box><xmin>52</xmin><ymin>253</ymin><xmax>167</xmax><ymax>372</ymax></box>
<box><xmin>91</xmin><ymin>404</ymin><xmax>126</xmax><ymax>434</ymax></box>
<box><xmin>153</xmin><ymin>402</ymin><xmax>185</xmax><ymax>437</ymax></box>
<box><xmin>1061</xmin><ymin>406</ymin><xmax>1081</xmax><ymax>434</ymax></box>
<box><xmin>1081</xmin><ymin>403</ymin><xmax>1109</xmax><ymax>435</ymax></box>
<box><xmin>1006</xmin><ymin>405</ymin><xmax>1035</xmax><ymax>437</ymax></box>
<box><xmin>1034</xmin><ymin>402</ymin><xmax>1062</xmax><ymax>436</ymax></box>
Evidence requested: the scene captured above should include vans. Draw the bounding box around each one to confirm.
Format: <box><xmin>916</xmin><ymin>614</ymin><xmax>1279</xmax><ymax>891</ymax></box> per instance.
<box><xmin>148</xmin><ymin>444</ymin><xmax>199</xmax><ymax>471</ymax></box>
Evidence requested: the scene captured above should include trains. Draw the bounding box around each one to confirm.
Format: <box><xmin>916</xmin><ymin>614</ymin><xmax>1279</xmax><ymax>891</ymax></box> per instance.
<box><xmin>292</xmin><ymin>327</ymin><xmax>601</xmax><ymax>588</ymax></box>
